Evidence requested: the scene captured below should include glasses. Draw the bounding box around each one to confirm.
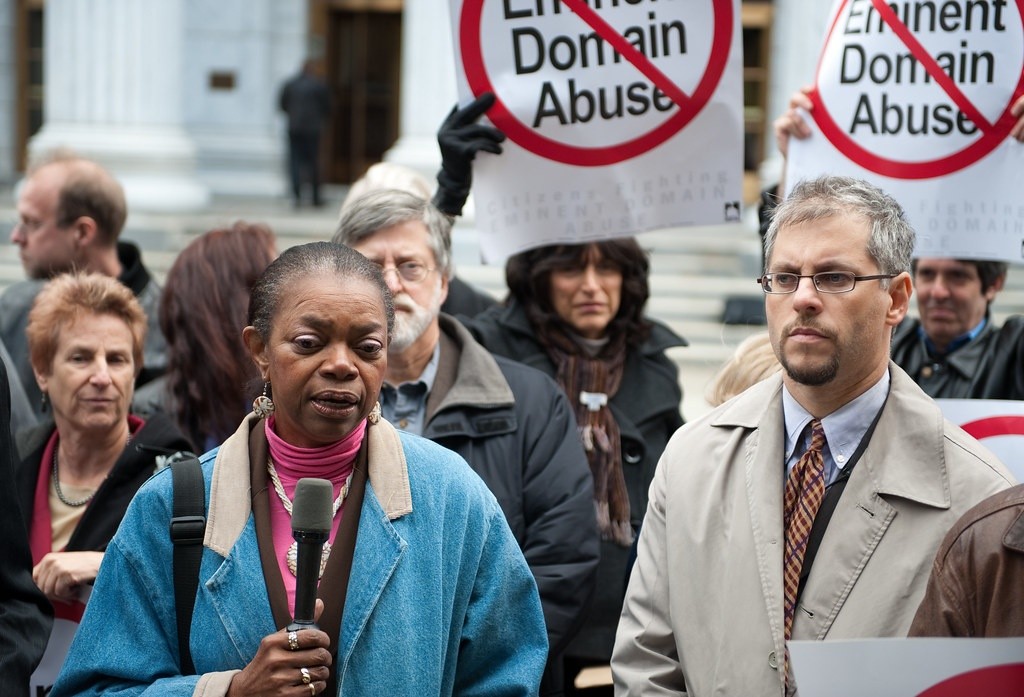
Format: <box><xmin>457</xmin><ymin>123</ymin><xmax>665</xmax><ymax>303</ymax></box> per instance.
<box><xmin>757</xmin><ymin>271</ymin><xmax>893</xmax><ymax>296</ymax></box>
<box><xmin>371</xmin><ymin>259</ymin><xmax>441</xmax><ymax>286</ymax></box>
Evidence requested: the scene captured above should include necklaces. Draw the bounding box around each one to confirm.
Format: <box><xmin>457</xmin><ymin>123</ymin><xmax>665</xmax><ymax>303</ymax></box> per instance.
<box><xmin>53</xmin><ymin>429</ymin><xmax>131</xmax><ymax>506</ymax></box>
<box><xmin>267</xmin><ymin>453</ymin><xmax>357</xmax><ymax>581</ymax></box>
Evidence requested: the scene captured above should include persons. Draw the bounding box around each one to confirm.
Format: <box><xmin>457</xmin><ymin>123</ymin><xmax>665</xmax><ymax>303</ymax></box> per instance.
<box><xmin>611</xmin><ymin>176</ymin><xmax>1021</xmax><ymax>697</ymax></box>
<box><xmin>0</xmin><ymin>80</ymin><xmax>689</xmax><ymax>697</ymax></box>
<box><xmin>280</xmin><ymin>57</ymin><xmax>334</xmax><ymax>209</ymax></box>
<box><xmin>705</xmin><ymin>82</ymin><xmax>1024</xmax><ymax>640</ymax></box>
<box><xmin>50</xmin><ymin>241</ymin><xmax>551</xmax><ymax>697</ymax></box>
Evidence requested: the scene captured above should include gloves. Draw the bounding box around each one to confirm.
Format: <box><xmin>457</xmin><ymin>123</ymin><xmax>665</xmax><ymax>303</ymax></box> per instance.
<box><xmin>432</xmin><ymin>91</ymin><xmax>507</xmax><ymax>220</ymax></box>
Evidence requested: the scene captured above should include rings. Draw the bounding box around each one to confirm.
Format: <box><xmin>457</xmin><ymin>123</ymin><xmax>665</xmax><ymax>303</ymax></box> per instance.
<box><xmin>309</xmin><ymin>683</ymin><xmax>316</xmax><ymax>696</ymax></box>
<box><xmin>288</xmin><ymin>631</ymin><xmax>300</xmax><ymax>650</ymax></box>
<box><xmin>300</xmin><ymin>667</ymin><xmax>310</xmax><ymax>684</ymax></box>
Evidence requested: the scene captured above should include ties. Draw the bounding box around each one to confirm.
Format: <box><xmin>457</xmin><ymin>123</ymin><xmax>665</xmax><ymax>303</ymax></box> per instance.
<box><xmin>774</xmin><ymin>420</ymin><xmax>828</xmax><ymax>640</ymax></box>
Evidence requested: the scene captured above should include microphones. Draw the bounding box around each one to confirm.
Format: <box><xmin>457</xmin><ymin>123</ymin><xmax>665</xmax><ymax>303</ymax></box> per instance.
<box><xmin>287</xmin><ymin>478</ymin><xmax>334</xmax><ymax>634</ymax></box>
